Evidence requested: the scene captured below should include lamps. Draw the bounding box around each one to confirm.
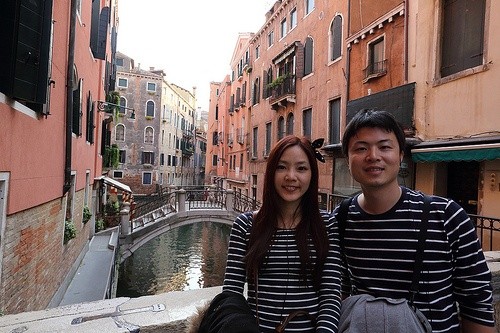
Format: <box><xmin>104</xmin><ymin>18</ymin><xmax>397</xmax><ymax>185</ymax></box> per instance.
<box><xmin>127</xmin><ymin>111</ymin><xmax>136</xmax><ymax>123</ymax></box>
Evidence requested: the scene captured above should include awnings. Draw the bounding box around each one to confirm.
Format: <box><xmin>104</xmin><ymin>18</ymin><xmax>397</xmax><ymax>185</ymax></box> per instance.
<box><xmin>103</xmin><ymin>176</ymin><xmax>132</xmax><ymax>193</ymax></box>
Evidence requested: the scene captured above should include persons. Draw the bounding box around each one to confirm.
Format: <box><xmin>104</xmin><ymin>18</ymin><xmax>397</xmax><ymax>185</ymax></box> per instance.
<box><xmin>222</xmin><ymin>134</ymin><xmax>343</xmax><ymax>333</ymax></box>
<box><xmin>329</xmin><ymin>109</ymin><xmax>498</xmax><ymax>333</ymax></box>
